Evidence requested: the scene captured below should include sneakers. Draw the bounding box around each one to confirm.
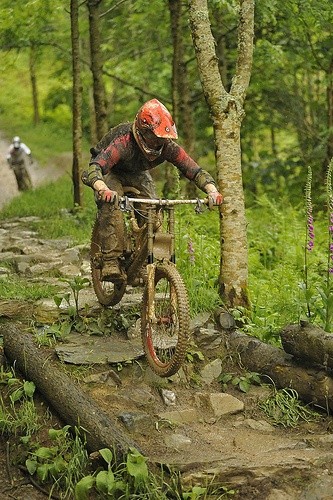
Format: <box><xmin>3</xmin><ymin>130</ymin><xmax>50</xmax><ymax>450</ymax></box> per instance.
<box><xmin>101</xmin><ymin>260</ymin><xmax>121</xmax><ymax>278</ymax></box>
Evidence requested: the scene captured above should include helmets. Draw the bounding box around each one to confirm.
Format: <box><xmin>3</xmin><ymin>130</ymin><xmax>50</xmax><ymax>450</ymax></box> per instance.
<box><xmin>134</xmin><ymin>99</ymin><xmax>178</xmax><ymax>155</ymax></box>
<box><xmin>13</xmin><ymin>136</ymin><xmax>20</xmax><ymax>149</ymax></box>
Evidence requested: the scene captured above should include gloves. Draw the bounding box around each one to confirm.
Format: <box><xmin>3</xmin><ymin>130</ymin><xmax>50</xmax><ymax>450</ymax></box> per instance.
<box><xmin>97</xmin><ymin>190</ymin><xmax>118</xmax><ymax>208</ymax></box>
<box><xmin>206</xmin><ymin>192</ymin><xmax>223</xmax><ymax>210</ymax></box>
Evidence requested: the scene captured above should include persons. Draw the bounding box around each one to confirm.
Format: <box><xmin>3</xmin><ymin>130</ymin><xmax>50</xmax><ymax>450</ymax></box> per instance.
<box><xmin>80</xmin><ymin>98</ymin><xmax>224</xmax><ymax>281</ymax></box>
<box><xmin>7</xmin><ymin>136</ymin><xmax>34</xmax><ymax>181</ymax></box>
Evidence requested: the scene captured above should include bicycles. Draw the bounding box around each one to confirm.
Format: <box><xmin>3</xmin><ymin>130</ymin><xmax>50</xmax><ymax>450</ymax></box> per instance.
<box><xmin>10</xmin><ymin>160</ymin><xmax>34</xmax><ymax>192</ymax></box>
<box><xmin>88</xmin><ymin>186</ymin><xmax>231</xmax><ymax>378</ymax></box>
